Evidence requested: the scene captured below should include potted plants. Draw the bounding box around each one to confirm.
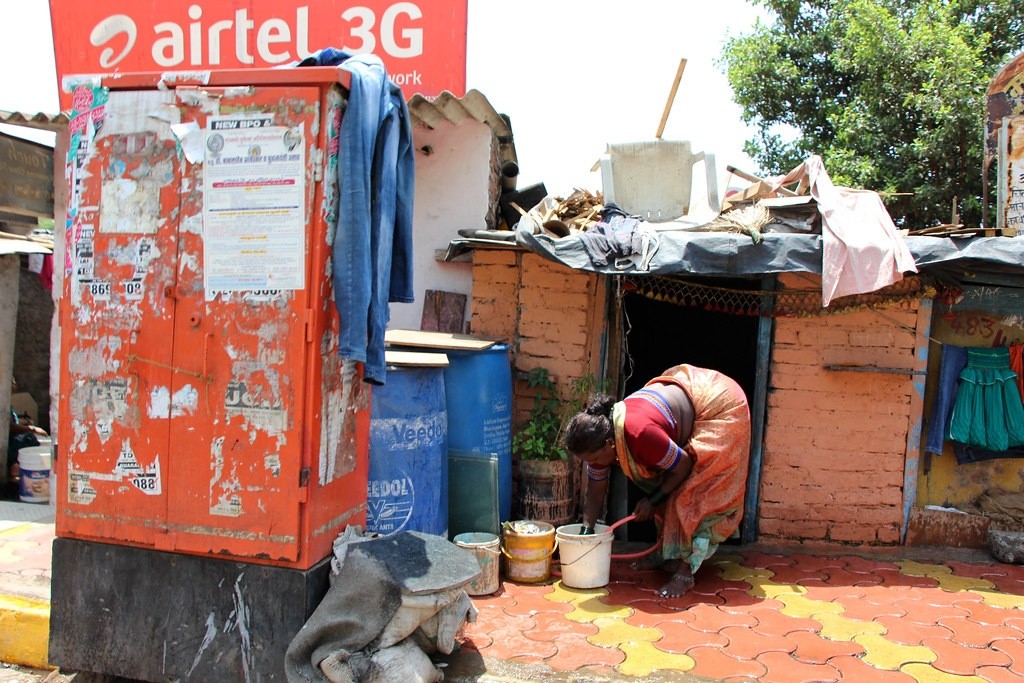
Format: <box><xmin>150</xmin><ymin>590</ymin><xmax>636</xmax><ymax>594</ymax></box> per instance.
<box><xmin>510</xmin><ymin>368</ymin><xmax>612</xmax><ymax>525</ymax></box>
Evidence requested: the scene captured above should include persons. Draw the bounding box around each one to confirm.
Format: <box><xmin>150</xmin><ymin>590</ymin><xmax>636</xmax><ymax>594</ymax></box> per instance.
<box><xmin>566</xmin><ymin>363</ymin><xmax>752</xmax><ymax>598</ymax></box>
<box><xmin>7</xmin><ymin>375</ymin><xmax>49</xmax><ymax>489</ymax></box>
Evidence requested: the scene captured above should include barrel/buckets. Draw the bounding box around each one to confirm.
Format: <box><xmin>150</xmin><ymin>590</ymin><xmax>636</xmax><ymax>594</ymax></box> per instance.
<box><xmin>554</xmin><ymin>523</ymin><xmax>614</xmax><ymax>588</ymax></box>
<box><xmin>365</xmin><ymin>362</ymin><xmax>450</xmax><ymax>538</ymax></box>
<box><xmin>454</xmin><ymin>532</ymin><xmax>501</xmax><ymax>596</ymax></box>
<box><xmin>18</xmin><ymin>446</ymin><xmax>51</xmax><ymax>502</ymax></box>
<box><xmin>501</xmin><ymin>520</ymin><xmax>557</xmax><ymax>583</ymax></box>
<box><xmin>390</xmin><ymin>343</ymin><xmax>513</xmax><ymax>522</ymax></box>
